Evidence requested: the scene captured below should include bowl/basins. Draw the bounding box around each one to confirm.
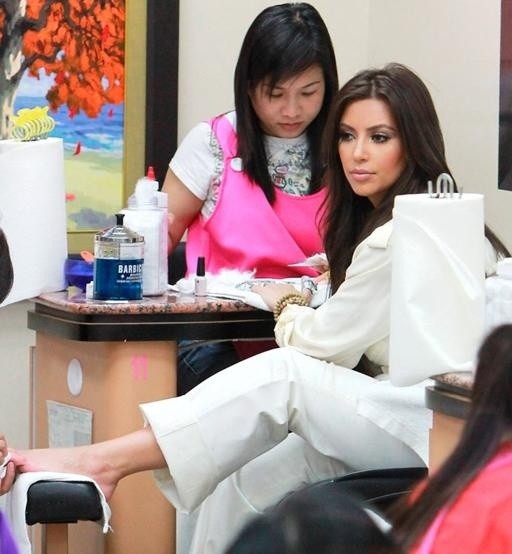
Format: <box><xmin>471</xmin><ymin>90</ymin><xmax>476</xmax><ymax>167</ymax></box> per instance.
<box><xmin>63</xmin><ymin>254</ymin><xmax>93</xmax><ymax>293</ymax></box>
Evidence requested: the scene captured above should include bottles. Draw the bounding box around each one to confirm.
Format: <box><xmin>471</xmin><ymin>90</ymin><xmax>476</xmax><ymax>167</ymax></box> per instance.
<box><xmin>196</xmin><ymin>256</ymin><xmax>207</xmax><ymax>297</ymax></box>
<box><xmin>122</xmin><ymin>166</ymin><xmax>170</xmax><ymax>297</ymax></box>
<box><xmin>91</xmin><ymin>212</ymin><xmax>145</xmax><ymax>302</ymax></box>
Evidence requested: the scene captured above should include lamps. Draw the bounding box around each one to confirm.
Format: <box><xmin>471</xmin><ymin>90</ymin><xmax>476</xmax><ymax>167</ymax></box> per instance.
<box><xmin>0</xmin><ymin>1</ymin><xmax>186</xmax><ymax>290</ymax></box>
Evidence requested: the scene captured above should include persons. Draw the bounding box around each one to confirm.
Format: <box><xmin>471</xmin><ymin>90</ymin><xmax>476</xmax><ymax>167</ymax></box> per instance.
<box><xmin>271</xmin><ymin>324</ymin><xmax>512</xmax><ymax>554</ymax></box>
<box><xmin>5</xmin><ymin>61</ymin><xmax>512</xmax><ymax>554</ymax></box>
<box><xmin>1</xmin><ymin>229</ymin><xmax>22</xmax><ymax>554</ymax></box>
<box><xmin>160</xmin><ymin>3</ymin><xmax>342</xmax><ymax>389</ymax></box>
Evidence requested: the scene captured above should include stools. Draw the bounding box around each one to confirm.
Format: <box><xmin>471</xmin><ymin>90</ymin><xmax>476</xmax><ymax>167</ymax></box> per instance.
<box><xmin>17</xmin><ymin>471</ymin><xmax>111</xmax><ymax>554</ymax></box>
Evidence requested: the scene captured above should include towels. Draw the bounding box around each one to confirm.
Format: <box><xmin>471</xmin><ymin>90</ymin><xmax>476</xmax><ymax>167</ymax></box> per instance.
<box><xmin>6</xmin><ymin>461</ymin><xmax>113</xmax><ymax>554</ymax></box>
<box><xmin>199</xmin><ymin>277</ymin><xmax>331</xmax><ymax>310</ymax></box>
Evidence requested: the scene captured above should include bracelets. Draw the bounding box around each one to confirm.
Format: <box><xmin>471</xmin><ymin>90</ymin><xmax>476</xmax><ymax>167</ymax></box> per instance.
<box><xmin>273</xmin><ymin>293</ymin><xmax>308</xmax><ymax>321</ymax></box>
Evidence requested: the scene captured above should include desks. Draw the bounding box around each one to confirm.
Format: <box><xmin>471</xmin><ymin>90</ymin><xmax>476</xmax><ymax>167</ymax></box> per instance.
<box><xmin>417</xmin><ymin>368</ymin><xmax>478</xmax><ymax>480</ymax></box>
<box><xmin>28</xmin><ymin>287</ymin><xmax>284</xmax><ymax>552</ymax></box>
